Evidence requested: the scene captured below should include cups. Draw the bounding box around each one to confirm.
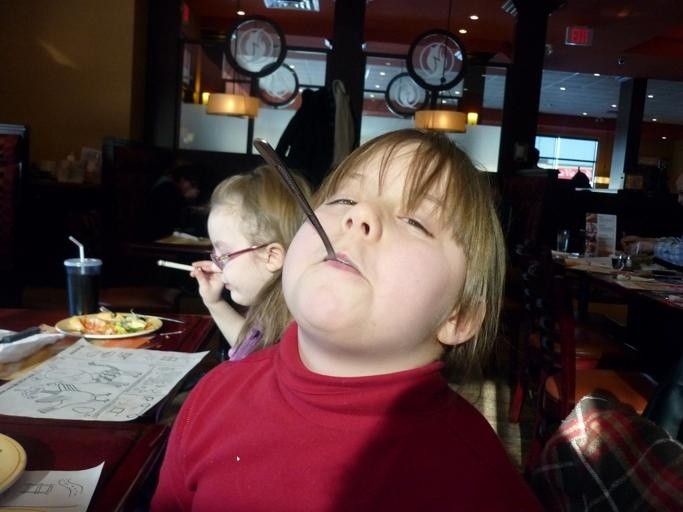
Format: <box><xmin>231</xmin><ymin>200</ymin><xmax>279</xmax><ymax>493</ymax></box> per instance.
<box><xmin>556</xmin><ymin>229</ymin><xmax>571</xmax><ymax>253</ymax></box>
<box><xmin>64</xmin><ymin>258</ymin><xmax>102</xmax><ymax>317</ymax></box>
<box><xmin>611</xmin><ymin>255</ymin><xmax>632</xmax><ymax>270</ymax></box>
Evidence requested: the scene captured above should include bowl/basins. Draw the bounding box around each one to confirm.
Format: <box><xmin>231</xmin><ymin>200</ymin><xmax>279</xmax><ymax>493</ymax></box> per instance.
<box><xmin>630</xmin><ymin>253</ymin><xmax>657</xmax><ymax>265</ymax></box>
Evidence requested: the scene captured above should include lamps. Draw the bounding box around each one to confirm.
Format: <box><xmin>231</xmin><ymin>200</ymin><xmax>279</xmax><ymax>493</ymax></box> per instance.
<box><xmin>202</xmin><ymin>0</ymin><xmax>261</xmax><ymax>119</ymax></box>
<box><xmin>414</xmin><ymin>2</ymin><xmax>467</xmax><ymax>135</ymax></box>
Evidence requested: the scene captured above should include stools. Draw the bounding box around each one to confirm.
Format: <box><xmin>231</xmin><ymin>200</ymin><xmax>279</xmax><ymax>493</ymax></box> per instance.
<box><xmin>98</xmin><ymin>284</ymin><xmax>182</xmax><ymax>315</ymax></box>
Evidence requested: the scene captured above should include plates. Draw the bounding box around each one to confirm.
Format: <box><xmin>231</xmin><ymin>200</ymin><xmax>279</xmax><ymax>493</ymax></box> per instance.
<box><xmin>1</xmin><ymin>433</ymin><xmax>27</xmax><ymax>494</ymax></box>
<box><xmin>55</xmin><ymin>310</ymin><xmax>164</xmax><ymax>340</ymax></box>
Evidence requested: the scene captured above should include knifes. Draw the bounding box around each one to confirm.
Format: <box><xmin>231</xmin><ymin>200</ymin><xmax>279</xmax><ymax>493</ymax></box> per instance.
<box><xmin>632</xmin><ymin>269</ymin><xmax>677</xmax><ymax>276</ymax></box>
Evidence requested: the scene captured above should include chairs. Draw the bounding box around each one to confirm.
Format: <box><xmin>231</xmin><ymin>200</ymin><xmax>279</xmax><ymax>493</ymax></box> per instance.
<box><xmin>500</xmin><ymin>211</ymin><xmax>682</xmax><ymax>511</ymax></box>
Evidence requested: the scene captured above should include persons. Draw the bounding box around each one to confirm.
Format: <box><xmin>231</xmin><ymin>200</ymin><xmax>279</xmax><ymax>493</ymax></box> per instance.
<box><xmin>619</xmin><ymin>171</ymin><xmax>683</xmax><ymax>266</ymax></box>
<box><xmin>147</xmin><ymin>126</ymin><xmax>543</xmax><ymax>511</ymax></box>
<box><xmin>190</xmin><ymin>163</ymin><xmax>312</xmax><ymax>362</ymax></box>
<box><xmin>482</xmin><ymin>174</ymin><xmax>525</xmax><ymax>296</ymax></box>
<box><xmin>163</xmin><ymin>169</ymin><xmax>209</xmax><ymax>303</ymax></box>
